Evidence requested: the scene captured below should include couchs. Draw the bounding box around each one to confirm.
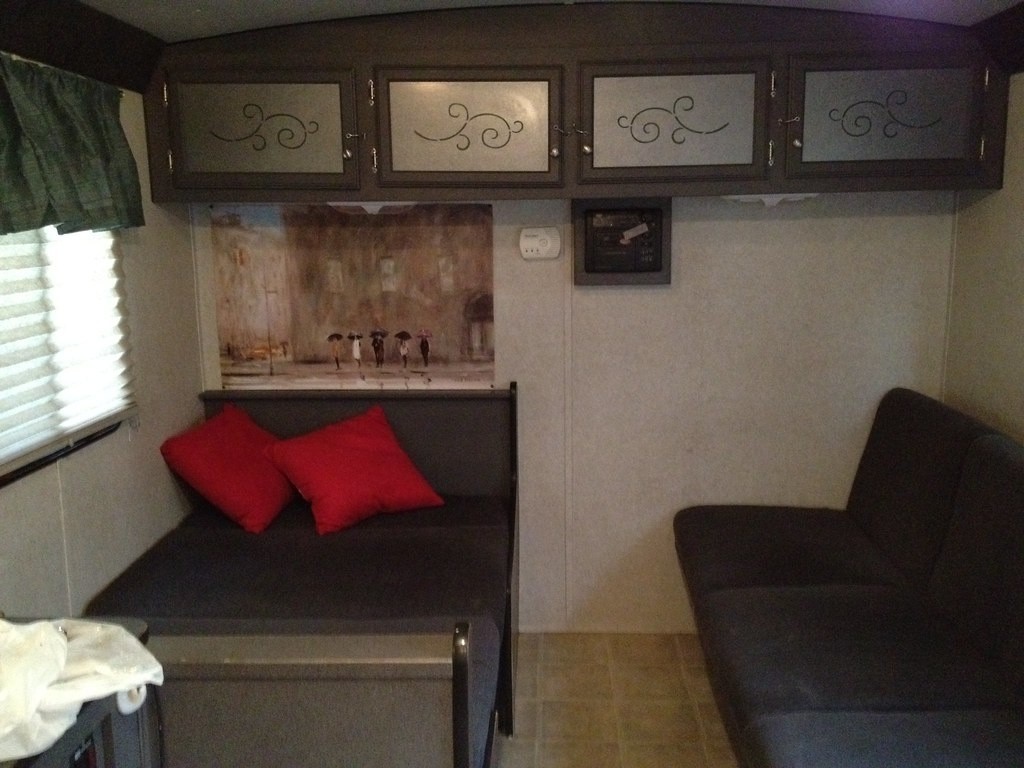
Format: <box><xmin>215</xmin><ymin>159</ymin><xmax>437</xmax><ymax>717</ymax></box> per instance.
<box><xmin>670</xmin><ymin>387</ymin><xmax>1024</xmax><ymax>768</ymax></box>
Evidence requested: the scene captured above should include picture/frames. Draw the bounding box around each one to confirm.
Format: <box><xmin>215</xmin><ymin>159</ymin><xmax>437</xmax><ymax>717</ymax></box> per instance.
<box><xmin>372</xmin><ymin>67</ymin><xmax>565</xmax><ymax>188</ymax></box>
<box><xmin>576</xmin><ymin>58</ymin><xmax>771</xmax><ymax>182</ymax></box>
<box><xmin>569</xmin><ymin>195</ymin><xmax>672</xmax><ymax>287</ymax></box>
<box><xmin>163</xmin><ymin>67</ymin><xmax>359</xmax><ymax>193</ymax></box>
<box><xmin>787</xmin><ymin>55</ymin><xmax>983</xmax><ymax>179</ymax></box>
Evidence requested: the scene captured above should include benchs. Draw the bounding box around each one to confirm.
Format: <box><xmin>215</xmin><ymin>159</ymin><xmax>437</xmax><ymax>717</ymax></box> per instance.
<box><xmin>82</xmin><ymin>382</ymin><xmax>521</xmax><ymax>768</ymax></box>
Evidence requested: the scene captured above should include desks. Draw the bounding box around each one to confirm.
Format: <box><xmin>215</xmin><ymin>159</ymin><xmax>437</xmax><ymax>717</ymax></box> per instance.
<box><xmin>0</xmin><ymin>615</ymin><xmax>149</xmax><ymax>768</ymax></box>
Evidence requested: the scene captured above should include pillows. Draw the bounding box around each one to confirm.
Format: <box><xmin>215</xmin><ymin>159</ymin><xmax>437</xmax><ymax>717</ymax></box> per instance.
<box><xmin>161</xmin><ymin>408</ymin><xmax>292</xmax><ymax>533</ymax></box>
<box><xmin>265</xmin><ymin>407</ymin><xmax>441</xmax><ymax>536</ymax></box>
